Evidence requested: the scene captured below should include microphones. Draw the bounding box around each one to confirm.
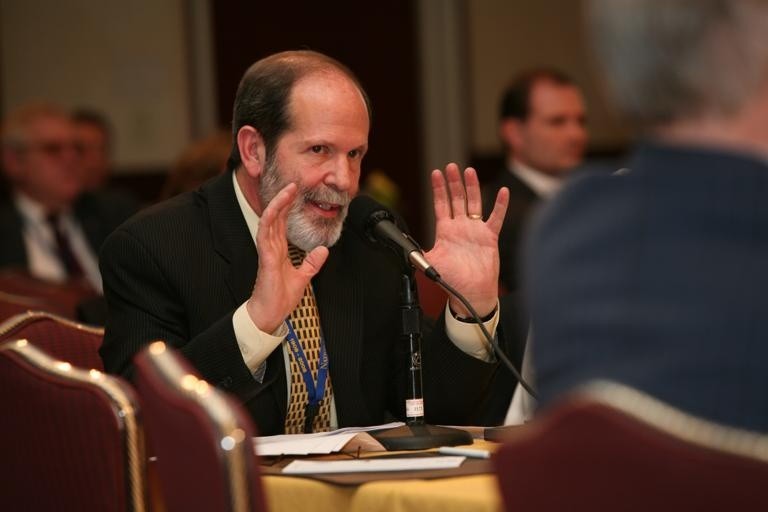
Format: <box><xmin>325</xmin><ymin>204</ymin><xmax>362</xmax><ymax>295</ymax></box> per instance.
<box><xmin>365</xmin><ymin>211</ymin><xmax>440</xmax><ymax>283</ymax></box>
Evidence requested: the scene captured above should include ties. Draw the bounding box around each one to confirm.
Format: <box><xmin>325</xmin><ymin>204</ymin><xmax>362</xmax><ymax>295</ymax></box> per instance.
<box><xmin>274</xmin><ymin>239</ymin><xmax>333</xmax><ymax>434</ymax></box>
<box><xmin>41</xmin><ymin>211</ymin><xmax>86</xmax><ymax>278</ymax></box>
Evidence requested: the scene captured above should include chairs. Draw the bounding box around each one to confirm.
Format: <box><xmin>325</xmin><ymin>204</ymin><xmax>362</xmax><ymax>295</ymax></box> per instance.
<box><xmin>2</xmin><ymin>264</ymin><xmax>271</xmax><ymax>512</ymax></box>
<box><xmin>488</xmin><ymin>378</ymin><xmax>768</xmax><ymax>512</ymax></box>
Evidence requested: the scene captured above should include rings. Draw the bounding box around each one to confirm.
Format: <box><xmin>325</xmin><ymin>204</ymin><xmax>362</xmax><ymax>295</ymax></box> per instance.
<box><xmin>468</xmin><ymin>214</ymin><xmax>484</xmax><ymax>219</ymax></box>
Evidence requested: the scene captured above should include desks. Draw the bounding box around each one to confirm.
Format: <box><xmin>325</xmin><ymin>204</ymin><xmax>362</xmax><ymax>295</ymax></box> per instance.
<box><xmin>253</xmin><ymin>424</ymin><xmax>501</xmax><ymax>512</ymax></box>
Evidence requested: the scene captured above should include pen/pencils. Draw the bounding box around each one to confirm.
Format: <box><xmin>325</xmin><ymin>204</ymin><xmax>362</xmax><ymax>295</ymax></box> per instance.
<box><xmin>440</xmin><ymin>446</ymin><xmax>490</xmax><ymax>459</ymax></box>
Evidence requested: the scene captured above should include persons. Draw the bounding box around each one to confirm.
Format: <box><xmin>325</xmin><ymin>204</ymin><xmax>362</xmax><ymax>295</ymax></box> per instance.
<box><xmin>1</xmin><ymin>101</ymin><xmax>115</xmax><ymax>299</ymax></box>
<box><xmin>96</xmin><ymin>50</ymin><xmax>512</xmax><ymax>442</ymax></box>
<box><xmin>482</xmin><ymin>66</ymin><xmax>611</xmax><ymax>302</ymax></box>
<box><xmin>74</xmin><ymin>105</ymin><xmax>113</xmax><ymax>194</ymax></box>
<box><xmin>521</xmin><ymin>1</ymin><xmax>767</xmax><ymax>436</ymax></box>
